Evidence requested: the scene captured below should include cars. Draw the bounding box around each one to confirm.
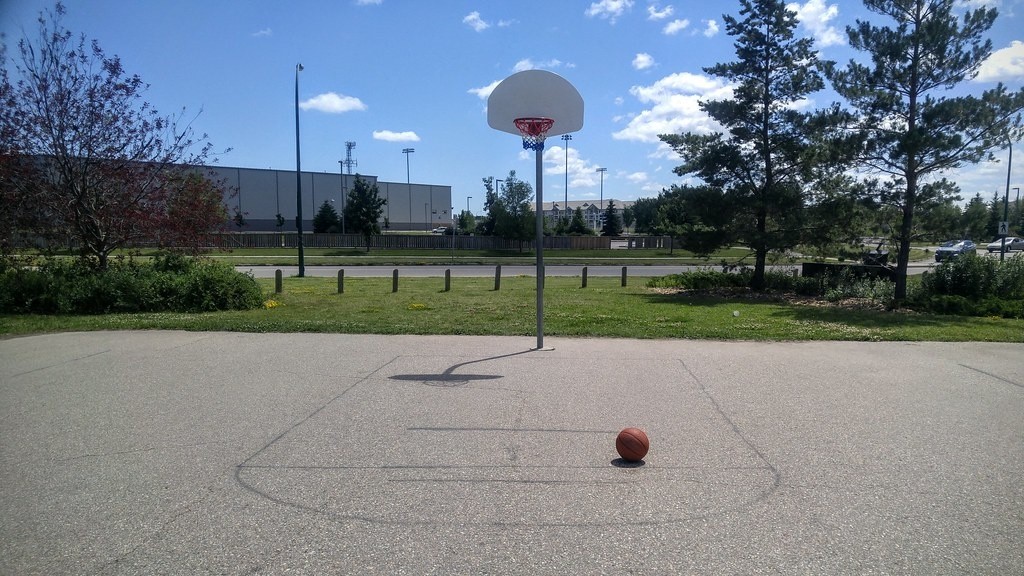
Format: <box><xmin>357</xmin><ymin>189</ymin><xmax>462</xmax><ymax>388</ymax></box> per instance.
<box><xmin>432</xmin><ymin>227</ymin><xmax>448</xmax><ymax>234</ymax></box>
<box><xmin>986</xmin><ymin>236</ymin><xmax>1024</xmax><ymax>251</ymax></box>
<box><xmin>935</xmin><ymin>240</ymin><xmax>977</xmax><ymax>262</ymax></box>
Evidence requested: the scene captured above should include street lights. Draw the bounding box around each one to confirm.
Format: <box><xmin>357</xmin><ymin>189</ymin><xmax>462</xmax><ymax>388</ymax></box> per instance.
<box><xmin>401</xmin><ymin>148</ymin><xmax>415</xmax><ymax>224</ymax></box>
<box><xmin>1012</xmin><ymin>187</ymin><xmax>1019</xmax><ymax>214</ymax></box>
<box><xmin>596</xmin><ymin>167</ymin><xmax>607</xmax><ymax>211</ymax></box>
<box><xmin>561</xmin><ymin>133</ymin><xmax>572</xmax><ymax>213</ymax></box>
<box><xmin>294</xmin><ymin>62</ymin><xmax>305</xmax><ymax>278</ymax></box>
<box><xmin>467</xmin><ymin>195</ymin><xmax>473</xmax><ymax>215</ymax></box>
<box><xmin>496</xmin><ymin>179</ymin><xmax>503</xmax><ymax>200</ymax></box>
<box><xmin>336</xmin><ymin>160</ymin><xmax>345</xmax><ymax>239</ymax></box>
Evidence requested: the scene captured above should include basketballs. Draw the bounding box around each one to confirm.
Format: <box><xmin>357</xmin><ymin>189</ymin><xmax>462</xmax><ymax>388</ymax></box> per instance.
<box><xmin>616</xmin><ymin>427</ymin><xmax>650</xmax><ymax>462</ymax></box>
